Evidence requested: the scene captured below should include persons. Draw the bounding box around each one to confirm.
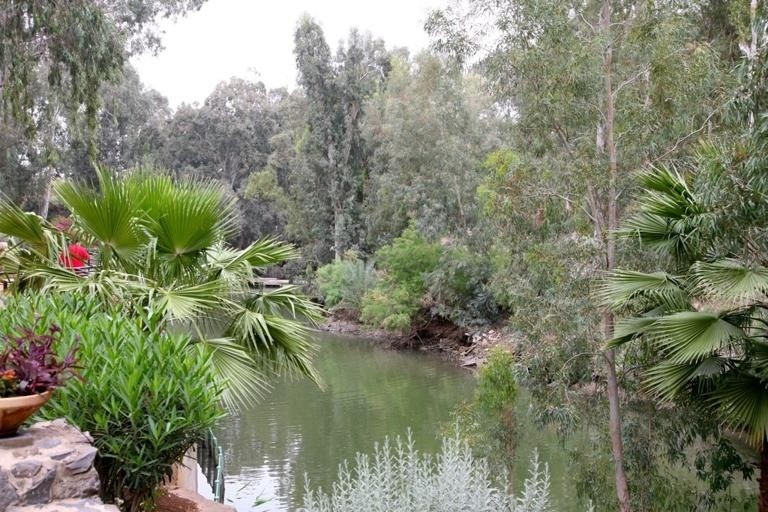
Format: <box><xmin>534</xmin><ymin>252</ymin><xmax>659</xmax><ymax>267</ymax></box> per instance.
<box><xmin>59</xmin><ymin>239</ymin><xmax>89</xmax><ymax>276</ymax></box>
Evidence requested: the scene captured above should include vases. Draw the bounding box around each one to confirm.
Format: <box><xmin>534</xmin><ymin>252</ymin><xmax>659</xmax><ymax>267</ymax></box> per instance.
<box><xmin>0</xmin><ymin>312</ymin><xmax>90</xmax><ymax>439</ymax></box>
